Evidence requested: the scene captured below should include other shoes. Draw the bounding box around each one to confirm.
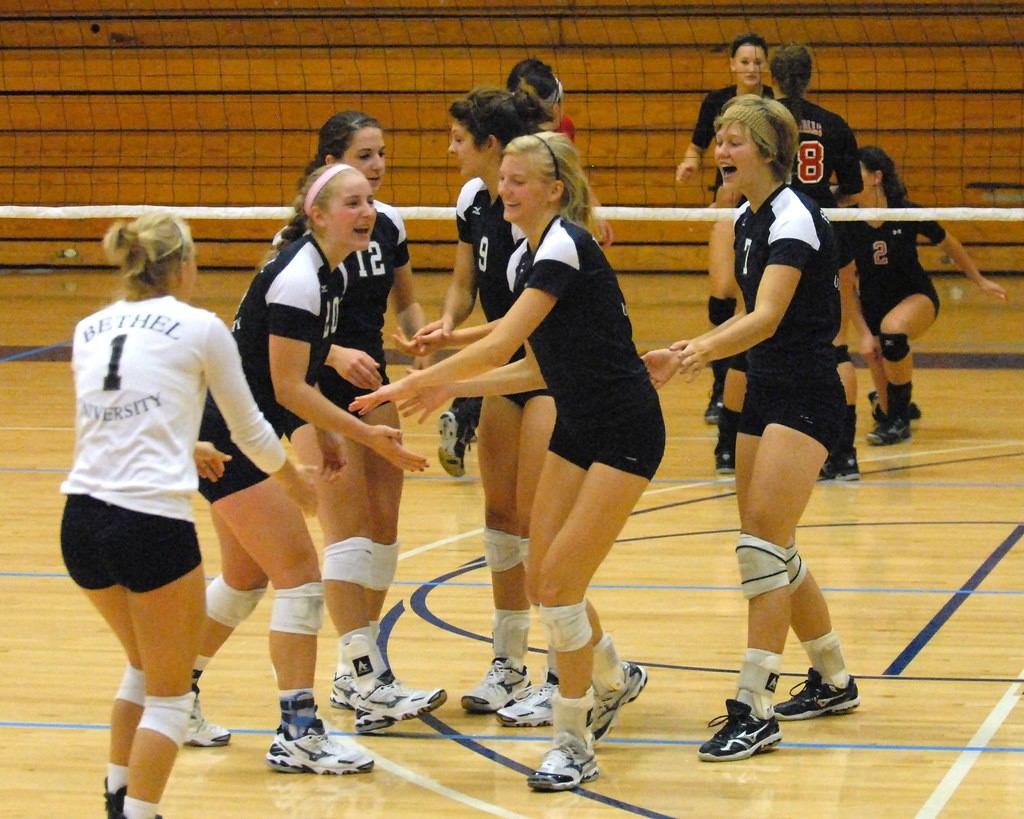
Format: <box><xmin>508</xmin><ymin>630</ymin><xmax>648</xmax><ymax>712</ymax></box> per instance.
<box><xmin>835</xmin><ymin>448</ymin><xmax>860</xmax><ymax>481</ymax></box>
<box><xmin>867</xmin><ymin>389</ymin><xmax>922</xmax><ymax>420</ymax></box>
<box><xmin>866</xmin><ymin>418</ymin><xmax>912</xmax><ymax>445</ymax></box>
<box><xmin>714</xmin><ymin>432</ymin><xmax>736</xmax><ymax>473</ymax></box>
<box><xmin>437</xmin><ymin>408</ymin><xmax>478</xmax><ymax>478</ymax></box>
<box><xmin>704</xmin><ymin>384</ymin><xmax>724</xmax><ymax>424</ymax></box>
<box><xmin>103</xmin><ymin>776</ymin><xmax>128</xmax><ymax>819</ymax></box>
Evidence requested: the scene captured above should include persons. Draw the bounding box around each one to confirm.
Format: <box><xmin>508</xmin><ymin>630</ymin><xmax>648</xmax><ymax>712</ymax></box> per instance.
<box><xmin>676</xmin><ymin>32</ymin><xmax>776</xmax><ymax>423</ymax></box>
<box><xmin>183</xmin><ymin>162</ymin><xmax>431</xmax><ymax>774</ymax></box>
<box><xmin>392</xmin><ymin>81</ymin><xmax>559</xmax><ymax>727</ymax></box>
<box><xmin>439</xmin><ymin>64</ymin><xmax>574</xmax><ymax>477</ymax></box>
<box><xmin>59</xmin><ymin>212</ymin><xmax>319</xmax><ymax>819</ymax></box>
<box><xmin>834</xmin><ymin>146</ymin><xmax>1009</xmax><ymax>444</ymax></box>
<box><xmin>640</xmin><ymin>94</ymin><xmax>860</xmax><ymax>763</ymax></box>
<box><xmin>258</xmin><ymin>112</ymin><xmax>448</xmax><ymax>731</ymax></box>
<box><xmin>347</xmin><ymin>132</ymin><xmax>666</xmax><ymax>790</ymax></box>
<box><xmin>715</xmin><ymin>40</ymin><xmax>864</xmax><ymax>478</ymax></box>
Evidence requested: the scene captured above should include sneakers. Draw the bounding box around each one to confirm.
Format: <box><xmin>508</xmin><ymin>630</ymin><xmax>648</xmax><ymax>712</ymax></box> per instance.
<box><xmin>496</xmin><ymin>683</ymin><xmax>595</xmax><ymax>727</ymax></box>
<box><xmin>527</xmin><ymin>741</ymin><xmax>600</xmax><ymax>790</ymax></box>
<box><xmin>330</xmin><ymin>673</ymin><xmax>357</xmax><ymax>710</ymax></box>
<box><xmin>773</xmin><ymin>667</ymin><xmax>861</xmax><ymax>721</ymax></box>
<box><xmin>355</xmin><ymin>684</ymin><xmax>448</xmax><ymax>732</ymax></box>
<box><xmin>587</xmin><ymin>659</ymin><xmax>649</xmax><ymax>746</ymax></box>
<box><xmin>264</xmin><ymin>718</ymin><xmax>374</xmax><ymax>775</ymax></box>
<box><xmin>461</xmin><ymin>655</ymin><xmax>534</xmax><ymax>712</ymax></box>
<box><xmin>186</xmin><ymin>692</ymin><xmax>232</xmax><ymax>747</ymax></box>
<box><xmin>697</xmin><ymin>699</ymin><xmax>783</xmax><ymax>762</ymax></box>
